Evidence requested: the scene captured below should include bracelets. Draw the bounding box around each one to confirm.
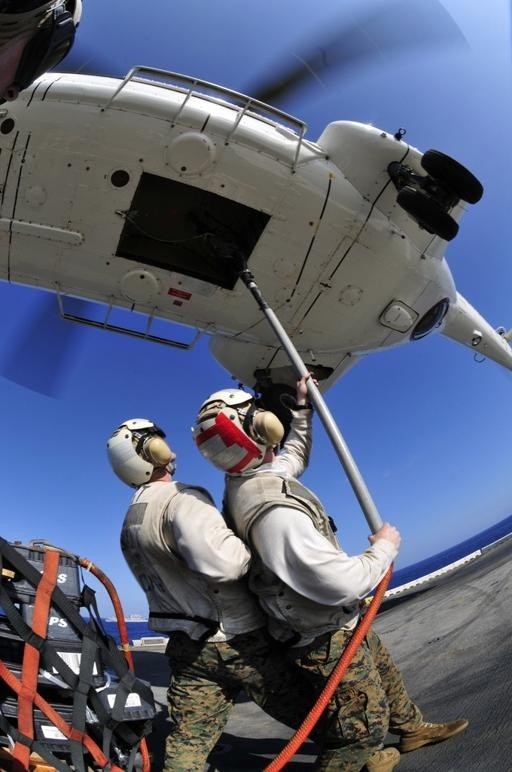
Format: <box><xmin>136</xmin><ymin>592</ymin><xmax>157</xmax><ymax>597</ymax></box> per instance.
<box><xmin>290</xmin><ymin>403</ymin><xmax>311</xmax><ymax>411</ymax></box>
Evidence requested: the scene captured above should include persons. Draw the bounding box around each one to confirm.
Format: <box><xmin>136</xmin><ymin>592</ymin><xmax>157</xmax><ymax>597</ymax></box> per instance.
<box><xmin>193</xmin><ymin>372</ymin><xmax>469</xmax><ymax>772</ymax></box>
<box><xmin>106</xmin><ymin>418</ymin><xmax>401</xmax><ymax>772</ymax></box>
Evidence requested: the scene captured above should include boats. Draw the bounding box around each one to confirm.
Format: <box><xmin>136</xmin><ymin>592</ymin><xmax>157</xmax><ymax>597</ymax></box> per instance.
<box><xmin>102</xmin><ymin>611</ymin><xmax>150</xmax><ymax>626</ymax></box>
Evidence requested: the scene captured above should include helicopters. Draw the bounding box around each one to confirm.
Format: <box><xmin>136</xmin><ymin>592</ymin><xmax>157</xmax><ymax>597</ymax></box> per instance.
<box><xmin>1</xmin><ymin>0</ymin><xmax>511</xmax><ymax>409</ymax></box>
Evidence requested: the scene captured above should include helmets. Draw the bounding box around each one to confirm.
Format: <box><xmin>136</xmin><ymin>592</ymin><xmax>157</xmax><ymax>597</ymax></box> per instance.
<box><xmin>106</xmin><ymin>417</ymin><xmax>176</xmax><ymax>488</ymax></box>
<box><xmin>192</xmin><ymin>388</ymin><xmax>287</xmax><ymax>473</ymax></box>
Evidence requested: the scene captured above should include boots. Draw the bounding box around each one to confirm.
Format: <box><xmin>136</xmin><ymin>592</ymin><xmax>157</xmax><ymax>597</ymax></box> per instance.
<box><xmin>401</xmin><ymin>718</ymin><xmax>471</xmax><ymax>755</ymax></box>
<box><xmin>366</xmin><ymin>746</ymin><xmax>401</xmax><ymax>772</ymax></box>
